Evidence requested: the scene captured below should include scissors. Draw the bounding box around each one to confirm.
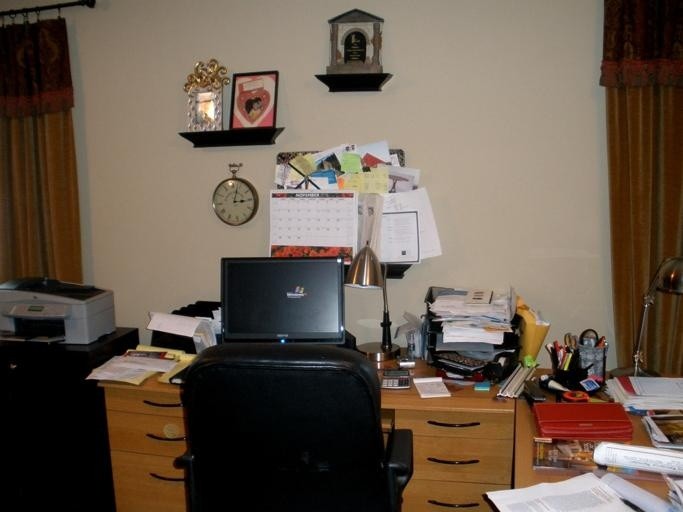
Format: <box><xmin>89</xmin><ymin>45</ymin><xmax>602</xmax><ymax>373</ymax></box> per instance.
<box><xmin>564</xmin><ymin>332</ymin><xmax>579</xmax><ymax>350</ymax></box>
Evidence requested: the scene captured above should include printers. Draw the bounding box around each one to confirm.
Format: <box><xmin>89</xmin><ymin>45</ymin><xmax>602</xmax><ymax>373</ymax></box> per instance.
<box><xmin>0</xmin><ymin>281</ymin><xmax>117</xmax><ymax>351</ymax></box>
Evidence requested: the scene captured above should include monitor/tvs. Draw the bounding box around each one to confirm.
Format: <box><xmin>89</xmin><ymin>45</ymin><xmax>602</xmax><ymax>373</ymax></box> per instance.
<box><xmin>220</xmin><ymin>255</ymin><xmax>346</xmax><ymax>345</ymax></box>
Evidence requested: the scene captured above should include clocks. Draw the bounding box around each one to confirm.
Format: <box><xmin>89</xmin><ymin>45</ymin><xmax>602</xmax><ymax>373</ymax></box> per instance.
<box><xmin>213</xmin><ymin>163</ymin><xmax>258</xmax><ymax>226</ymax></box>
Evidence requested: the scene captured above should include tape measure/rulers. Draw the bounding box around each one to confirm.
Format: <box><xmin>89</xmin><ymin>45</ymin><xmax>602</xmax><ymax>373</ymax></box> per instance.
<box><xmin>562</xmin><ymin>391</ymin><xmax>591</xmax><ymax>402</ymax></box>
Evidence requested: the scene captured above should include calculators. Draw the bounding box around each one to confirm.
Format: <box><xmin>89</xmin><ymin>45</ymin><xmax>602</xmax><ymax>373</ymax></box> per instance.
<box><xmin>380</xmin><ymin>369</ymin><xmax>410</xmax><ymax>389</ymax></box>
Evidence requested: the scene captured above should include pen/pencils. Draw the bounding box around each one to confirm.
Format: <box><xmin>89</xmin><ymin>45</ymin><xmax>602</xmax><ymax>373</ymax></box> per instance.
<box><xmin>545</xmin><ymin>341</ymin><xmax>574</xmax><ymax>371</ymax></box>
<box><xmin>621</xmin><ymin>497</ymin><xmax>650</xmax><ymax>512</ymax></box>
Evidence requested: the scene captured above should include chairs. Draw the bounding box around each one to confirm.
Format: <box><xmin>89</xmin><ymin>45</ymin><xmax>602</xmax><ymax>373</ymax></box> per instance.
<box><xmin>168</xmin><ymin>346</ymin><xmax>413</xmax><ymax>512</ymax></box>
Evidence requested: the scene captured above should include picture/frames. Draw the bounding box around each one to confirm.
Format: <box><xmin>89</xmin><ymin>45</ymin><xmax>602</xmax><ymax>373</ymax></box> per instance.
<box><xmin>230</xmin><ymin>70</ymin><xmax>279</xmax><ymax>128</ymax></box>
<box><xmin>184</xmin><ymin>59</ymin><xmax>229</xmax><ymax>132</ymax></box>
<box><xmin>326</xmin><ymin>10</ymin><xmax>384</xmax><ymax>74</ymax></box>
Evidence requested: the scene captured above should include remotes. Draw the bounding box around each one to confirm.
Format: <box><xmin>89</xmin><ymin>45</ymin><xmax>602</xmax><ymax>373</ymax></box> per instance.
<box><xmin>55</xmin><ymin>284</ymin><xmax>94</xmax><ymax>294</ymax></box>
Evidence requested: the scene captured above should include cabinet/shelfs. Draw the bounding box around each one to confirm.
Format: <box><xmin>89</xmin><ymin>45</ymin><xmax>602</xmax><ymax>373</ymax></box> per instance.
<box><xmin>0</xmin><ymin>327</ymin><xmax>139</xmax><ymax>511</ymax></box>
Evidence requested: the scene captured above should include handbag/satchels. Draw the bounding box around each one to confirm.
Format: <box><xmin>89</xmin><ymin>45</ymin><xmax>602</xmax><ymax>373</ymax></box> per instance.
<box><xmin>530</xmin><ymin>400</ymin><xmax>633</xmax><ymax>441</ymax></box>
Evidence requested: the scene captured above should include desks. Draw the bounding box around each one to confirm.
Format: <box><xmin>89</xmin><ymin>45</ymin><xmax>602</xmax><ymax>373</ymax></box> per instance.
<box><xmin>516</xmin><ymin>367</ymin><xmax>669</xmax><ymax>502</ymax></box>
<box><xmin>98</xmin><ymin>357</ymin><xmax>515</xmax><ymax>512</ymax></box>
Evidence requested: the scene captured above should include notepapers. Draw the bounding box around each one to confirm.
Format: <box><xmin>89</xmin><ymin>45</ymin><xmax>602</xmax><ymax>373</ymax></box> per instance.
<box><xmin>474</xmin><ymin>382</ymin><xmax>491</xmax><ymax>392</ymax></box>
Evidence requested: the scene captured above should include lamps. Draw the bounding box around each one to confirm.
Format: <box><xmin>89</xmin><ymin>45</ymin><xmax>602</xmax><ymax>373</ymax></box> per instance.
<box><xmin>633</xmin><ymin>254</ymin><xmax>682</xmax><ymax>375</ymax></box>
<box><xmin>344</xmin><ymin>240</ymin><xmax>401</xmax><ymax>363</ymax></box>
